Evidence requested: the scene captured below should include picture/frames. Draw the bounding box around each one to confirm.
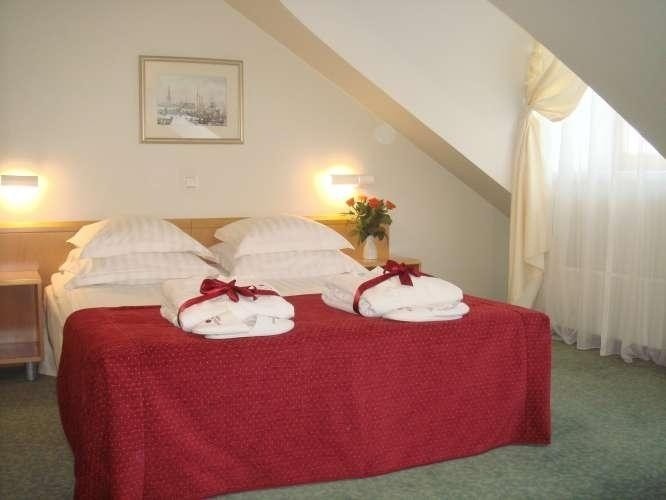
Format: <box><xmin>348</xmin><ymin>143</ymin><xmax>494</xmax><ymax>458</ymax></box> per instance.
<box><xmin>138</xmin><ymin>54</ymin><xmax>246</xmax><ymax>145</ymax></box>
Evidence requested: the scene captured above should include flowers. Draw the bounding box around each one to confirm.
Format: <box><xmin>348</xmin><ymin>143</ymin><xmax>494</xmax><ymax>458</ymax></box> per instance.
<box><xmin>346</xmin><ymin>193</ymin><xmax>396</xmax><ymax>244</ymax></box>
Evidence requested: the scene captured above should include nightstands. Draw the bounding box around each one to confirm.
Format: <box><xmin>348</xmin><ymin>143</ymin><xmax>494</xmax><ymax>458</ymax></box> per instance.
<box><xmin>1</xmin><ymin>272</ymin><xmax>47</xmax><ymax>384</ymax></box>
<box><xmin>361</xmin><ymin>257</ymin><xmax>425</xmax><ymax>274</ymax></box>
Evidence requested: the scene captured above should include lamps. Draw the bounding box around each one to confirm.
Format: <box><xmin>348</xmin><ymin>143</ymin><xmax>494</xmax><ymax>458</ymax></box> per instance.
<box><xmin>0</xmin><ymin>173</ymin><xmax>40</xmax><ymax>192</ymax></box>
<box><xmin>330</xmin><ymin>173</ymin><xmax>375</xmax><ymax>189</ymax></box>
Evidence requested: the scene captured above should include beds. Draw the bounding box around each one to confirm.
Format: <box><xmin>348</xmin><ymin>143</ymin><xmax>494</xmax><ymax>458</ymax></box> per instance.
<box><xmin>0</xmin><ymin>213</ymin><xmax>551</xmax><ymax>500</ymax></box>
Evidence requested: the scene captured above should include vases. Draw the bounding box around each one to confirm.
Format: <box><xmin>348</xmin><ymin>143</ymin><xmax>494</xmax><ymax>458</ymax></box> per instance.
<box><xmin>360</xmin><ymin>233</ymin><xmax>379</xmax><ymax>261</ymax></box>
